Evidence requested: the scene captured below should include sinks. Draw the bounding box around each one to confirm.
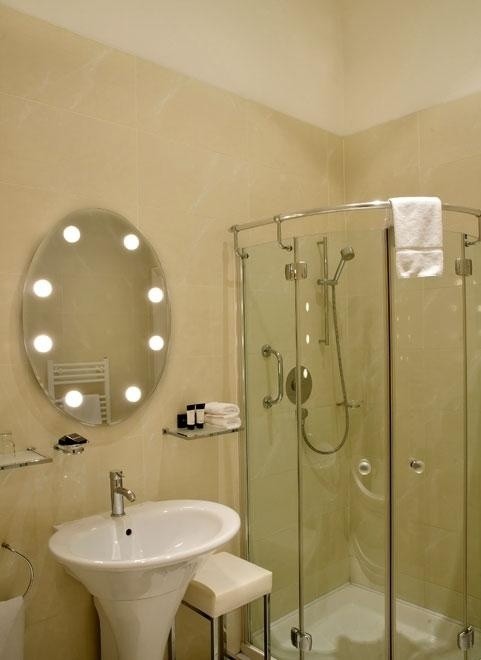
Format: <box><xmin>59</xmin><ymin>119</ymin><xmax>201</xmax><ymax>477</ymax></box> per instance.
<box><xmin>49</xmin><ymin>496</ymin><xmax>241</xmax><ymax>602</ymax></box>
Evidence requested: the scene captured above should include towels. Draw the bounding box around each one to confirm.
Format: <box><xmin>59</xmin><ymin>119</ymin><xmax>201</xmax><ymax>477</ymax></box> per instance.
<box><xmin>204</xmin><ymin>418</ymin><xmax>241</xmax><ymax>429</ymax></box>
<box><xmin>384</xmin><ymin>197</ymin><xmax>445</xmax><ymax>279</ymax></box>
<box><xmin>1</xmin><ymin>596</ymin><xmax>25</xmax><ymax>660</ymax></box>
<box><xmin>204</xmin><ymin>401</ymin><xmax>240</xmax><ymax>419</ymax></box>
<box><xmin>63</xmin><ymin>394</ymin><xmax>101</xmax><ymax>425</ymax></box>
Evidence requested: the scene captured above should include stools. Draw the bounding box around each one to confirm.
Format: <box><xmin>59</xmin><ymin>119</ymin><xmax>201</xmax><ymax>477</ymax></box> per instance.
<box><xmin>168</xmin><ymin>552</ymin><xmax>273</xmax><ymax>660</ymax></box>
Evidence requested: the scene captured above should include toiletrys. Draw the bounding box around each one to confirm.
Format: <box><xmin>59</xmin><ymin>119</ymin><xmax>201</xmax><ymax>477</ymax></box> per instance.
<box><xmin>186</xmin><ymin>404</ymin><xmax>199</xmax><ymax>429</ymax></box>
<box><xmin>197</xmin><ymin>402</ymin><xmax>207</xmax><ymax>431</ymax></box>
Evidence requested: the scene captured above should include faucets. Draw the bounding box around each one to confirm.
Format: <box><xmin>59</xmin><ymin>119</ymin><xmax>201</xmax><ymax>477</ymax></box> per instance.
<box><xmin>107</xmin><ymin>468</ymin><xmax>136</xmax><ymax>518</ymax></box>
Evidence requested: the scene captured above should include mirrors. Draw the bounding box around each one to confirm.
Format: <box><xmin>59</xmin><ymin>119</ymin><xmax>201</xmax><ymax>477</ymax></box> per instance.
<box><xmin>23</xmin><ymin>208</ymin><xmax>171</xmax><ymax>426</ymax></box>
